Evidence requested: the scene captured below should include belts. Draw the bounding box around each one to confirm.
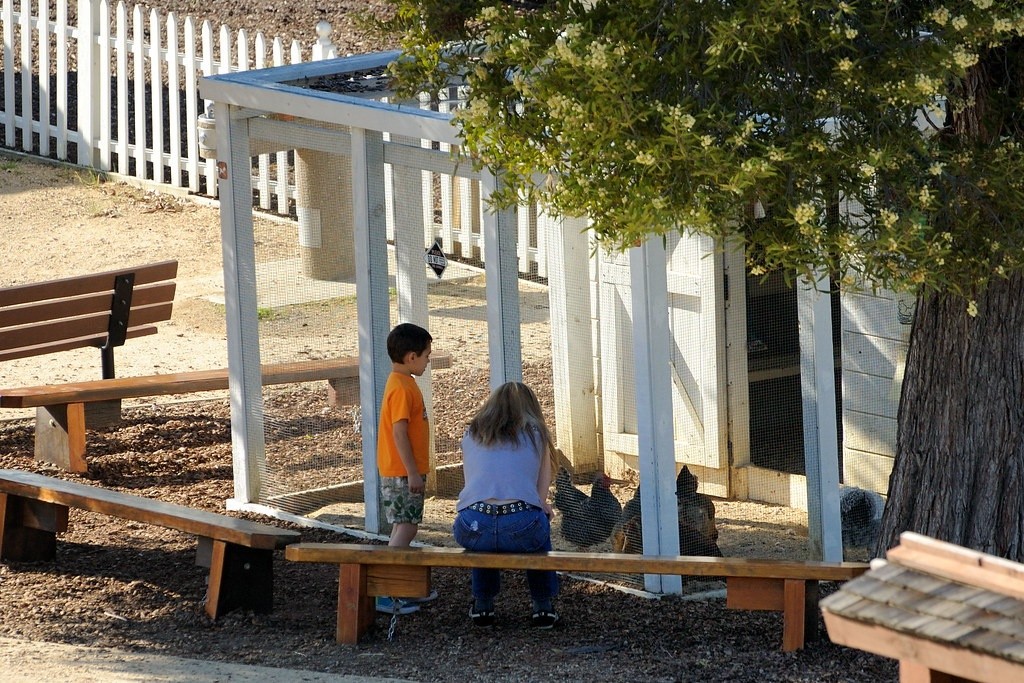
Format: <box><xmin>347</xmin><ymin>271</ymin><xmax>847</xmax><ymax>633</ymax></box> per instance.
<box><xmin>468</xmin><ymin>497</ymin><xmax>533</xmax><ymax>516</ymax></box>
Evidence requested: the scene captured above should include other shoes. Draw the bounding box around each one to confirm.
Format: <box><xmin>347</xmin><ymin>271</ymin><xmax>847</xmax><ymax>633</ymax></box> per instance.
<box><xmin>532</xmin><ymin>608</ymin><xmax>558</xmax><ymax>628</ymax></box>
<box><xmin>468</xmin><ymin>601</ymin><xmax>494</xmax><ymax>628</ymax></box>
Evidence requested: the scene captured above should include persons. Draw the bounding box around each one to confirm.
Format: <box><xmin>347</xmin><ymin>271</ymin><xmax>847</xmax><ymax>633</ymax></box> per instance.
<box><xmin>375</xmin><ymin>323</ymin><xmax>437</xmax><ymax>614</ymax></box>
<box><xmin>452</xmin><ymin>381</ymin><xmax>560</xmax><ymax>629</ymax></box>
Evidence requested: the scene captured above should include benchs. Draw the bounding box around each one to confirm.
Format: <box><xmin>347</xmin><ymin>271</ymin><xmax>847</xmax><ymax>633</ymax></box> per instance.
<box><xmin>0</xmin><ymin>352</ymin><xmax>452</xmax><ymax>472</ymax></box>
<box><xmin>0</xmin><ymin>259</ymin><xmax>179</xmax><ymax>379</ymax></box>
<box><xmin>286</xmin><ymin>543</ymin><xmax>871</xmax><ymax>654</ymax></box>
<box><xmin>0</xmin><ymin>468</ymin><xmax>302</xmax><ymax>621</ymax></box>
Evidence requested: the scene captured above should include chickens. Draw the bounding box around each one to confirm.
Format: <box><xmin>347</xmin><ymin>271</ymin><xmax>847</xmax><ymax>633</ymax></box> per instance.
<box><xmin>840</xmin><ymin>485</ymin><xmax>885</xmax><ymax>562</ymax></box>
<box><xmin>553</xmin><ymin>463</ymin><xmax>728</xmax><ymax>585</ymax></box>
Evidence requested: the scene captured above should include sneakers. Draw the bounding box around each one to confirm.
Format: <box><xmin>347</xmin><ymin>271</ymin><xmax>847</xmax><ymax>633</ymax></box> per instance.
<box><xmin>401</xmin><ymin>590</ymin><xmax>438</xmax><ymax>602</ymax></box>
<box><xmin>375</xmin><ymin>595</ymin><xmax>419</xmax><ymax>614</ymax></box>
